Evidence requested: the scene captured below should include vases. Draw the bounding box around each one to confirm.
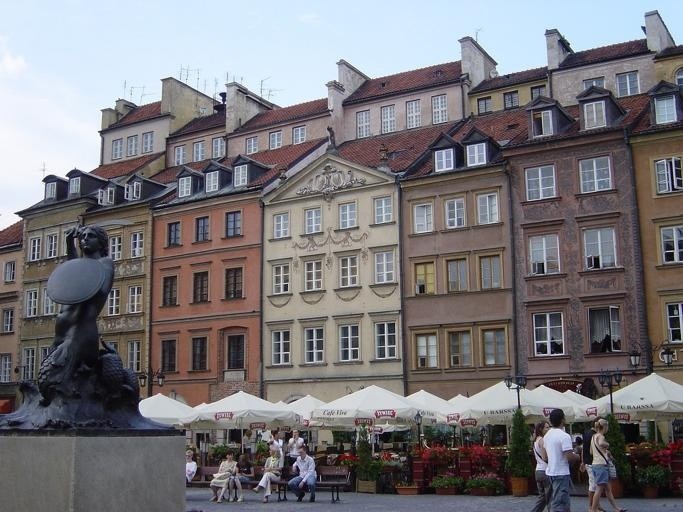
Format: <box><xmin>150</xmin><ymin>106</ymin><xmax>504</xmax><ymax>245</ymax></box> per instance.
<box><xmin>436</xmin><ymin>487</ymin><xmax>458</xmax><ymax>495</ymax></box>
<box><xmin>472</xmin><ymin>488</ymin><xmax>494</xmax><ymax>495</ymax></box>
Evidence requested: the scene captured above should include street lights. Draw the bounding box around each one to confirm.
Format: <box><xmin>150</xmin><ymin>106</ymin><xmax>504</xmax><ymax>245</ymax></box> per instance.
<box><xmin>598</xmin><ymin>368</ymin><xmax>623</xmax><ymax>413</ymax></box>
<box><xmin>504</xmin><ymin>372</ymin><xmax>527</xmax><ymax>409</ymax></box>
<box><xmin>138</xmin><ymin>366</ymin><xmax>164</xmax><ymax>398</ymax></box>
<box><xmin>413</xmin><ymin>411</ymin><xmax>422</xmax><ymax>447</ymax></box>
<box><xmin>628</xmin><ymin>339</ymin><xmax>675</xmax><ymax>377</ymax></box>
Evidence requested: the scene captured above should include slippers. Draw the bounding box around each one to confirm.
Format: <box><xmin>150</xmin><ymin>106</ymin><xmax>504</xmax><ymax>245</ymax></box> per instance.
<box><xmin>619</xmin><ymin>507</ymin><xmax>628</xmax><ymax>512</ymax></box>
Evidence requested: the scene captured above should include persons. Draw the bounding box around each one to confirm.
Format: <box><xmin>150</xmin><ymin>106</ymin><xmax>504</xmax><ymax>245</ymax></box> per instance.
<box><xmin>288</xmin><ymin>446</ymin><xmax>316</xmax><ymax>502</ymax></box>
<box><xmin>529</xmin><ymin>420</ymin><xmax>552</xmax><ymax>512</ymax></box>
<box><xmin>186</xmin><ymin>450</ymin><xmax>197</xmax><ymax>484</ymax></box>
<box><xmin>252</xmin><ymin>447</ymin><xmax>283</xmax><ymax>503</ymax></box>
<box><xmin>543</xmin><ymin>408</ymin><xmax>581</xmax><ymax>512</ymax></box>
<box><xmin>580</xmin><ymin>416</ymin><xmax>607</xmax><ymax>512</ymax></box>
<box><xmin>242</xmin><ymin>428</ymin><xmax>305</xmax><ymax>466</ymax></box>
<box><xmin>40</xmin><ymin>224</ymin><xmax>115</xmax><ymax>370</ymax></box>
<box><xmin>590</xmin><ymin>419</ymin><xmax>628</xmax><ymax>512</ymax></box>
<box><xmin>228</xmin><ymin>455</ymin><xmax>254</xmax><ymax>502</ymax></box>
<box><xmin>209</xmin><ymin>449</ymin><xmax>238</xmax><ymax>503</ymax></box>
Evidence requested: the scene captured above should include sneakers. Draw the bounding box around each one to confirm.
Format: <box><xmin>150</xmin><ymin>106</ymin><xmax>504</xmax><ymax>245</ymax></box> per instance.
<box><xmin>253</xmin><ymin>488</ymin><xmax>259</xmax><ymax>493</ymax></box>
<box><xmin>209</xmin><ymin>495</ymin><xmax>243</xmax><ymax>503</ymax></box>
<box><xmin>297</xmin><ymin>492</ymin><xmax>305</xmax><ymax>502</ymax></box>
<box><xmin>263</xmin><ymin>499</ymin><xmax>268</xmax><ymax>503</ymax></box>
<box><xmin>309</xmin><ymin>494</ymin><xmax>315</xmax><ymax>502</ymax></box>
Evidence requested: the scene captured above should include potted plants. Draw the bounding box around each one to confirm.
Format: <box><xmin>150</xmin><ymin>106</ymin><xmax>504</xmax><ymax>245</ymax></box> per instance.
<box><xmin>394</xmin><ymin>455</ymin><xmax>421</xmax><ymax>495</ymax></box>
<box><xmin>506</xmin><ymin>408</ymin><xmax>534</xmax><ymax>496</ymax></box>
<box><xmin>355</xmin><ymin>427</ymin><xmax>377</xmax><ymax>494</ymax></box>
<box><xmin>603</xmin><ymin>413</ymin><xmax>627</xmax><ymax>499</ymax></box>
<box><xmin>635</xmin><ymin>463</ymin><xmax>666</xmax><ymax>498</ymax></box>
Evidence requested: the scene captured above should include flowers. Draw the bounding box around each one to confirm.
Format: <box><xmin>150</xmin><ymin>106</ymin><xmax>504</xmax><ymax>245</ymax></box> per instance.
<box><xmin>465</xmin><ymin>472</ymin><xmax>505</xmax><ymax>490</ymax></box>
<box><xmin>431</xmin><ymin>471</ymin><xmax>462</xmax><ymax>488</ymax></box>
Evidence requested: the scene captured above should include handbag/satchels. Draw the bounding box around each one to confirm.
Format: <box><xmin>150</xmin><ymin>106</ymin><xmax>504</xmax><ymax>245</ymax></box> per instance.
<box><xmin>213</xmin><ymin>472</ymin><xmax>230</xmax><ymax>480</ymax></box>
<box><xmin>609</xmin><ymin>464</ymin><xmax>617</xmax><ymax>480</ymax></box>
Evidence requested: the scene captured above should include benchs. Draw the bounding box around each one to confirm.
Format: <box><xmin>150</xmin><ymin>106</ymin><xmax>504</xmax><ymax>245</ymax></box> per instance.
<box><xmin>185</xmin><ymin>466</ymin><xmax>351</xmax><ymax>503</ymax></box>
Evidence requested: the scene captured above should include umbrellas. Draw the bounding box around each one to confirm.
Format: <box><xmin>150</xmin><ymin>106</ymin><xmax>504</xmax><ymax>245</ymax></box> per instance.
<box><xmin>586</xmin><ymin>372</ymin><xmax>683</xmax><ymax>446</ymax></box>
